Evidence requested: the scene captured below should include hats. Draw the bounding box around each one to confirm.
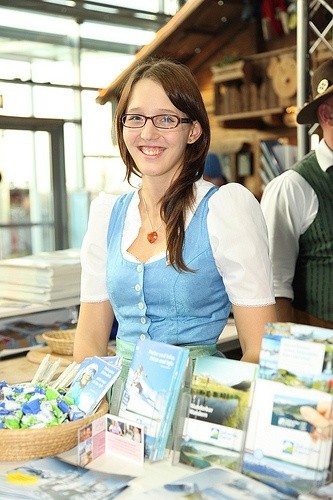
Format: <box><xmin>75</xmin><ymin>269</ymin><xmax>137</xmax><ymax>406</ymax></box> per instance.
<box><xmin>296</xmin><ymin>59</ymin><xmax>333</xmax><ymax>125</ymax></box>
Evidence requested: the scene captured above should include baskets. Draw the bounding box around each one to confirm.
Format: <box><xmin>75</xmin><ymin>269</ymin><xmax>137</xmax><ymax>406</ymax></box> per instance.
<box><xmin>41</xmin><ymin>329</ymin><xmax>76</xmax><ymax>355</ymax></box>
<box><xmin>0</xmin><ymin>390</ymin><xmax>108</xmax><ymax>462</ymax></box>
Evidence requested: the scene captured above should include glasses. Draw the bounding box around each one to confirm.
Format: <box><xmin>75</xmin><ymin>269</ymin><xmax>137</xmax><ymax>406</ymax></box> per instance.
<box><xmin>119</xmin><ymin>114</ymin><xmax>191</xmax><ymax>129</ymax></box>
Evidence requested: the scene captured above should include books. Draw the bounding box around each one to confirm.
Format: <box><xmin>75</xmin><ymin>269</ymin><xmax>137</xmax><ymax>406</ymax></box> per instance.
<box><xmin>0</xmin><ymin>246</ymin><xmax>81</xmax><ymax>309</ymax></box>
<box><xmin>251</xmin><ymin>135</ymin><xmax>301</xmax><ymax>196</ymax></box>
<box><xmin>0</xmin><ymin>322</ymin><xmax>333</xmax><ymax>500</ymax></box>
<box><xmin>207</xmin><ymin>55</ymin><xmax>286</xmax><ymax>118</ymax></box>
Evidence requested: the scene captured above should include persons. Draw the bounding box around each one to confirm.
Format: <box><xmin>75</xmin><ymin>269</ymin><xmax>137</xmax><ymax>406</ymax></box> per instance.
<box><xmin>259</xmin><ymin>58</ymin><xmax>333</xmax><ymax>329</ymax></box>
<box><xmin>73</xmin><ymin>60</ymin><xmax>278</xmax><ymax>365</ymax></box>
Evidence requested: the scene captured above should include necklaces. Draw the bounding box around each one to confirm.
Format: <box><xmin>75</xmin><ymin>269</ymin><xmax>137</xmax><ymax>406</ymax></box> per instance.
<box><xmin>140</xmin><ymin>195</ymin><xmax>169</xmax><ymax>244</ymax></box>
